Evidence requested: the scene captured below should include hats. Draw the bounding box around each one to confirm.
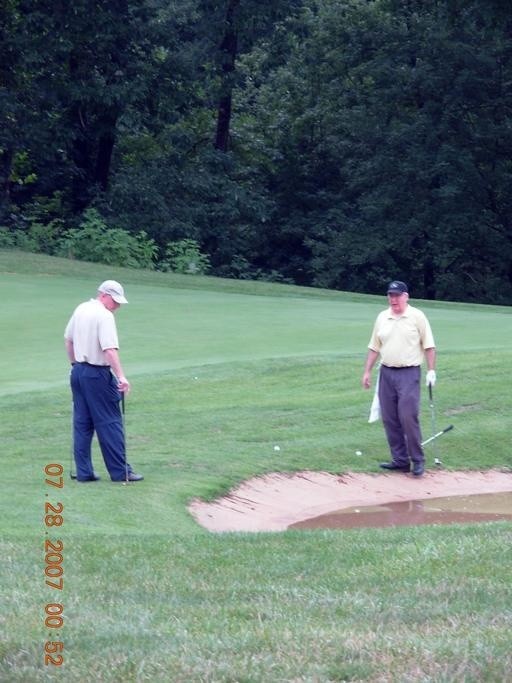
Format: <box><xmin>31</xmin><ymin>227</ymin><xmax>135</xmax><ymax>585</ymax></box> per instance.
<box><xmin>386</xmin><ymin>281</ymin><xmax>408</xmax><ymax>295</ymax></box>
<box><xmin>97</xmin><ymin>279</ymin><xmax>129</xmax><ymax>305</ymax></box>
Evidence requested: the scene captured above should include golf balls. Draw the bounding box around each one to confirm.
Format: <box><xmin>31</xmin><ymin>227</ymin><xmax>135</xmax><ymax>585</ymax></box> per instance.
<box><xmin>356</xmin><ymin>451</ymin><xmax>361</xmax><ymax>455</ymax></box>
<box><xmin>274</xmin><ymin>445</ymin><xmax>280</xmax><ymax>451</ymax></box>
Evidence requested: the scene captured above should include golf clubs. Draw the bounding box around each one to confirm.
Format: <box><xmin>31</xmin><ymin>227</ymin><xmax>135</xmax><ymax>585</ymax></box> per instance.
<box><xmin>70</xmin><ymin>396</ymin><xmax>77</xmax><ymax>478</ymax></box>
<box><xmin>121</xmin><ymin>391</ymin><xmax>129</xmax><ymax>486</ymax></box>
<box><xmin>428</xmin><ymin>382</ymin><xmax>442</xmax><ymax>465</ymax></box>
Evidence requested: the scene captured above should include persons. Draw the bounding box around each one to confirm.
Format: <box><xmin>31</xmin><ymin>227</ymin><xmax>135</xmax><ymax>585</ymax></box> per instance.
<box><xmin>64</xmin><ymin>279</ymin><xmax>144</xmax><ymax>482</ymax></box>
<box><xmin>362</xmin><ymin>279</ymin><xmax>436</xmax><ymax>474</ymax></box>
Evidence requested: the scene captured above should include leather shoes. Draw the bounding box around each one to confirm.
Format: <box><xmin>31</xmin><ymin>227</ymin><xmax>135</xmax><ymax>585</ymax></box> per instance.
<box><xmin>413</xmin><ymin>462</ymin><xmax>425</xmax><ymax>476</ymax></box>
<box><xmin>379</xmin><ymin>461</ymin><xmax>411</xmax><ymax>473</ymax></box>
<box><xmin>124</xmin><ymin>471</ymin><xmax>144</xmax><ymax>482</ymax></box>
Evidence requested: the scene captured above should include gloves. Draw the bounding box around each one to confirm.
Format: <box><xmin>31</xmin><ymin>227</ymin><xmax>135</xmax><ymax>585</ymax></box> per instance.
<box><xmin>426</xmin><ymin>370</ymin><xmax>436</xmax><ymax>387</ymax></box>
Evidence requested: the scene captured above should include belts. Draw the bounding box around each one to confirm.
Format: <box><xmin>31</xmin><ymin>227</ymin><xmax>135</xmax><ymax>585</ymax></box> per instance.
<box><xmin>83</xmin><ymin>362</ymin><xmax>110</xmax><ymax>369</ymax></box>
